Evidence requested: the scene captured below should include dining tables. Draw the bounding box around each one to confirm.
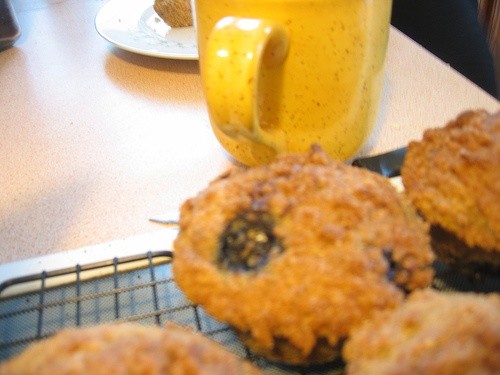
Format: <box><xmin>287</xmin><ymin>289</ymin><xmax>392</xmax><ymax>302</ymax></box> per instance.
<box><xmin>0</xmin><ymin>0</ymin><xmax>500</xmax><ymax>268</ymax></box>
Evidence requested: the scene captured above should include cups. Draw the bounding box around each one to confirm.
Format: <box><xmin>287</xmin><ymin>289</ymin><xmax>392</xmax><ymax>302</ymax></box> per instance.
<box><xmin>196</xmin><ymin>0</ymin><xmax>393</xmax><ymax>167</ymax></box>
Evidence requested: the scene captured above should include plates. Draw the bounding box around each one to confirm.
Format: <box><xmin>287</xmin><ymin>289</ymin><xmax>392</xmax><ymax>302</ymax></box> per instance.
<box><xmin>94</xmin><ymin>0</ymin><xmax>199</xmax><ymax>60</ymax></box>
